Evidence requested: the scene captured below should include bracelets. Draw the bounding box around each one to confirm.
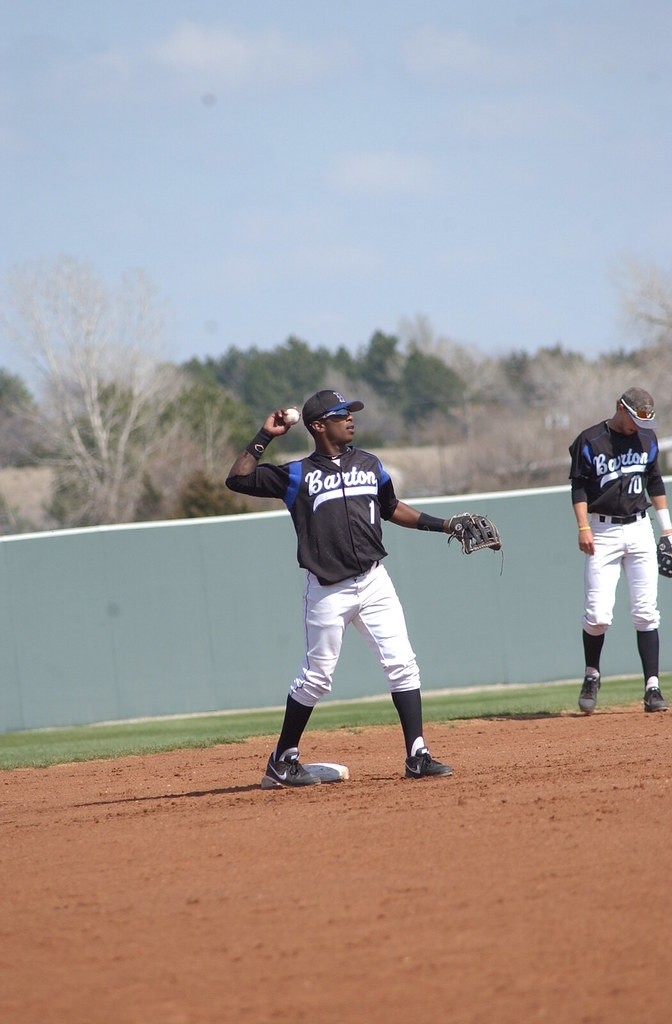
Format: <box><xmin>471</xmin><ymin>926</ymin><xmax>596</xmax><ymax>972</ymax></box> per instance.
<box><xmin>578</xmin><ymin>526</ymin><xmax>591</xmax><ymax>532</ymax></box>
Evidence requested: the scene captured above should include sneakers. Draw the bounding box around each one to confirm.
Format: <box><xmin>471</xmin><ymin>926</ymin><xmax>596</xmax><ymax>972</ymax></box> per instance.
<box><xmin>643</xmin><ymin>686</ymin><xmax>669</xmax><ymax>712</ymax></box>
<box><xmin>264</xmin><ymin>752</ymin><xmax>321</xmax><ymax>787</ymax></box>
<box><xmin>578</xmin><ymin>674</ymin><xmax>601</xmax><ymax>714</ymax></box>
<box><xmin>405</xmin><ymin>747</ymin><xmax>452</xmax><ymax>778</ymax></box>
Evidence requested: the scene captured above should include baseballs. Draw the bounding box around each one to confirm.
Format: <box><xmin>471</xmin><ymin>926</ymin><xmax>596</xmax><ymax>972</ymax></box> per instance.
<box><xmin>282</xmin><ymin>408</ymin><xmax>299</xmax><ymax>424</ymax></box>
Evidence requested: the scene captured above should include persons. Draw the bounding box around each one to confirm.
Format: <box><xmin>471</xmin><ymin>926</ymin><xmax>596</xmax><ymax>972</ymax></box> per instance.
<box><xmin>224</xmin><ymin>388</ymin><xmax>502</xmax><ymax>791</ymax></box>
<box><xmin>568</xmin><ymin>385</ymin><xmax>672</xmax><ymax>714</ymax></box>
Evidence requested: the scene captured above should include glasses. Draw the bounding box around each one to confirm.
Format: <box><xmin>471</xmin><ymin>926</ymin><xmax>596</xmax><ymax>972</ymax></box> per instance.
<box><xmin>309</xmin><ymin>409</ymin><xmax>351</xmax><ymax>426</ymax></box>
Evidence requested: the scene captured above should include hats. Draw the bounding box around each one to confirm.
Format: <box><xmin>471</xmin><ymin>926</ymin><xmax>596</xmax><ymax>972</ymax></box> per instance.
<box><xmin>302</xmin><ymin>390</ymin><xmax>364</xmax><ymax>426</ymax></box>
<box><xmin>620</xmin><ymin>387</ymin><xmax>658</xmax><ymax>429</ymax></box>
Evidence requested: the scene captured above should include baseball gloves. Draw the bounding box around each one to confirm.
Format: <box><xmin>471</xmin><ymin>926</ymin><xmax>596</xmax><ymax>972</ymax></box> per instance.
<box><xmin>447</xmin><ymin>514</ymin><xmax>500</xmax><ymax>554</ymax></box>
<box><xmin>657</xmin><ymin>534</ymin><xmax>672</xmax><ymax>577</ymax></box>
